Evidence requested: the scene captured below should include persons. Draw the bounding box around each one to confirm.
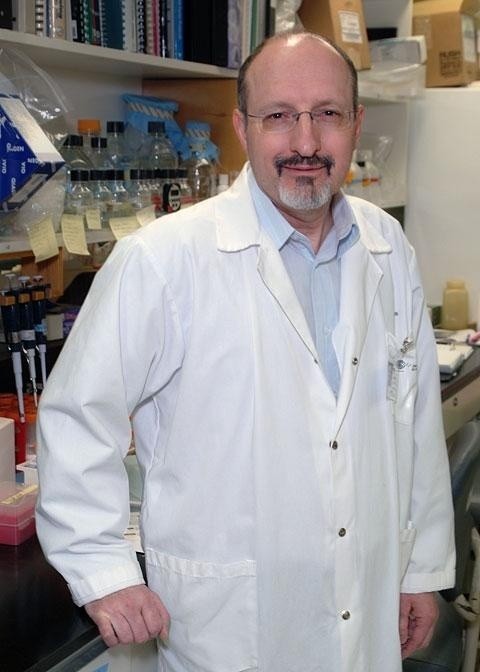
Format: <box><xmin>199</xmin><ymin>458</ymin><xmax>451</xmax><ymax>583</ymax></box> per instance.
<box><xmin>31</xmin><ymin>29</ymin><xmax>461</xmax><ymax>671</ymax></box>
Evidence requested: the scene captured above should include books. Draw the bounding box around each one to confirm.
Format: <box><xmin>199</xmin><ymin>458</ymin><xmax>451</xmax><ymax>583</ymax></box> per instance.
<box><xmin>0</xmin><ymin>1</ymin><xmax>276</xmax><ymax>72</ymax></box>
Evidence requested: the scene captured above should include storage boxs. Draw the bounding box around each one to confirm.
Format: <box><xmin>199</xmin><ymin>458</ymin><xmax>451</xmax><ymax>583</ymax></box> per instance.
<box><xmin>410</xmin><ymin>0</ymin><xmax>480</xmax><ymax>90</ymax></box>
<box><xmin>298</xmin><ymin>0</ymin><xmax>370</xmax><ymax>73</ymax></box>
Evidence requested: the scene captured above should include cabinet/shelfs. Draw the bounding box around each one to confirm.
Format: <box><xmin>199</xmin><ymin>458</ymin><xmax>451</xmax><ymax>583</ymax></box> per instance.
<box><xmin>1</xmin><ymin>2</ymin><xmax>410</xmax><ymax>306</ymax></box>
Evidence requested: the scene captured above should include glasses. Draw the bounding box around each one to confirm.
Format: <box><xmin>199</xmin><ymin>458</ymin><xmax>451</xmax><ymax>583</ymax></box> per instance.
<box><xmin>245</xmin><ymin>105</ymin><xmax>359</xmax><ymax>137</ymax></box>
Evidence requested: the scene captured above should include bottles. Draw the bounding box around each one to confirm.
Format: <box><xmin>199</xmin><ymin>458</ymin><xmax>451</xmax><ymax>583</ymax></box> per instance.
<box><xmin>337</xmin><ymin>136</ymin><xmax>397</xmax><ymax>207</ymax></box>
<box><xmin>26</xmin><ymin>413</ymin><xmax>39</xmax><ymax>468</ymax></box>
<box><xmin>60</xmin><ymin>114</ymin><xmax>225</xmax><ymax>230</ymax></box>
<box><xmin>438</xmin><ymin>275</ymin><xmax>470</xmax><ymax>331</ymax></box>
<box><xmin>1</xmin><ymin>268</ymin><xmax>60</xmax><ymax>343</ymax></box>
